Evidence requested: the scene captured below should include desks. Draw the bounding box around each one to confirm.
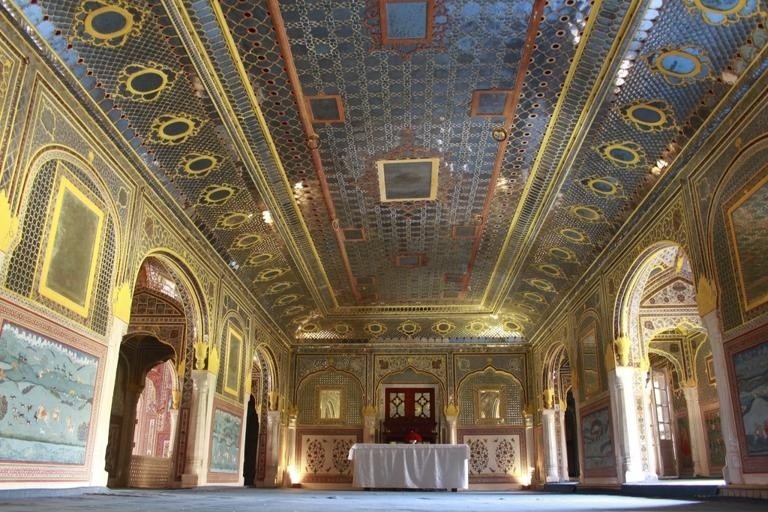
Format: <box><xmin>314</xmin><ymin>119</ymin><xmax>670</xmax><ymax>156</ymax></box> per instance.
<box><xmin>352</xmin><ymin>442</ymin><xmax>468</xmax><ymax>493</ymax></box>
